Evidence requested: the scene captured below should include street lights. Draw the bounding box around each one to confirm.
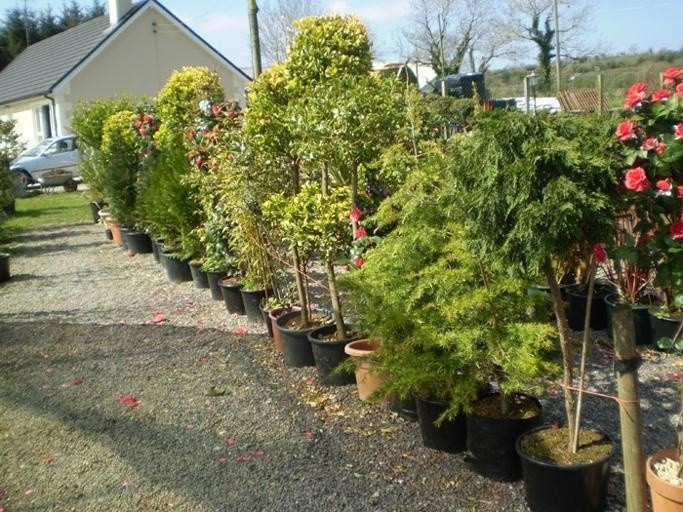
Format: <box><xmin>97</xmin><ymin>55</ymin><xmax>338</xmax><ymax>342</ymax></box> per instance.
<box><xmin>525</xmin><ymin>69</ymin><xmax>540</xmax><ymax>114</ymax></box>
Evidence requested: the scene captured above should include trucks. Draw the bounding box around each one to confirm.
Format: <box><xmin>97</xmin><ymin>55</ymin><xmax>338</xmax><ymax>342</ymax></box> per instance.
<box><xmin>438</xmin><ymin>73</ymin><xmax>518</xmax><ymax>115</ymax></box>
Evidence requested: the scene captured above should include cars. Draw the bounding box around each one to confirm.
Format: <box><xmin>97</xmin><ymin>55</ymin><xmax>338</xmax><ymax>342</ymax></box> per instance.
<box><xmin>8</xmin><ymin>133</ymin><xmax>82</xmax><ymax>187</ymax></box>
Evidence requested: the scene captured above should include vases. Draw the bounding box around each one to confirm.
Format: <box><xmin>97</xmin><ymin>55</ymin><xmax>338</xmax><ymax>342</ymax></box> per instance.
<box><xmin>644</xmin><ymin>447</ymin><xmax>683</xmax><ymax>512</ymax></box>
<box><xmin>528</xmin><ymin>273</ymin><xmax>683</xmax><ymax>348</ymax></box>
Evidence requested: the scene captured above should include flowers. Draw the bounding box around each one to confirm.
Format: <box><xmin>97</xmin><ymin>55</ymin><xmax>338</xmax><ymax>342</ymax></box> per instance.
<box><xmin>614</xmin><ymin>67</ymin><xmax>683</xmax><ymax>314</ymax></box>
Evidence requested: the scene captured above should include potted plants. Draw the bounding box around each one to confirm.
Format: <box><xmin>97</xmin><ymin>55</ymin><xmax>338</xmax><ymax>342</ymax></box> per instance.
<box><xmin>72</xmin><ymin>66</ymin><xmax>218</xmax><ymax>299</ymax></box>
<box><xmin>449</xmin><ymin>99</ymin><xmax>611</xmax><ymax>510</ymax></box>
<box><xmin>0</xmin><ymin>118</ymin><xmax>22</xmax><ymax>283</ymax></box>
